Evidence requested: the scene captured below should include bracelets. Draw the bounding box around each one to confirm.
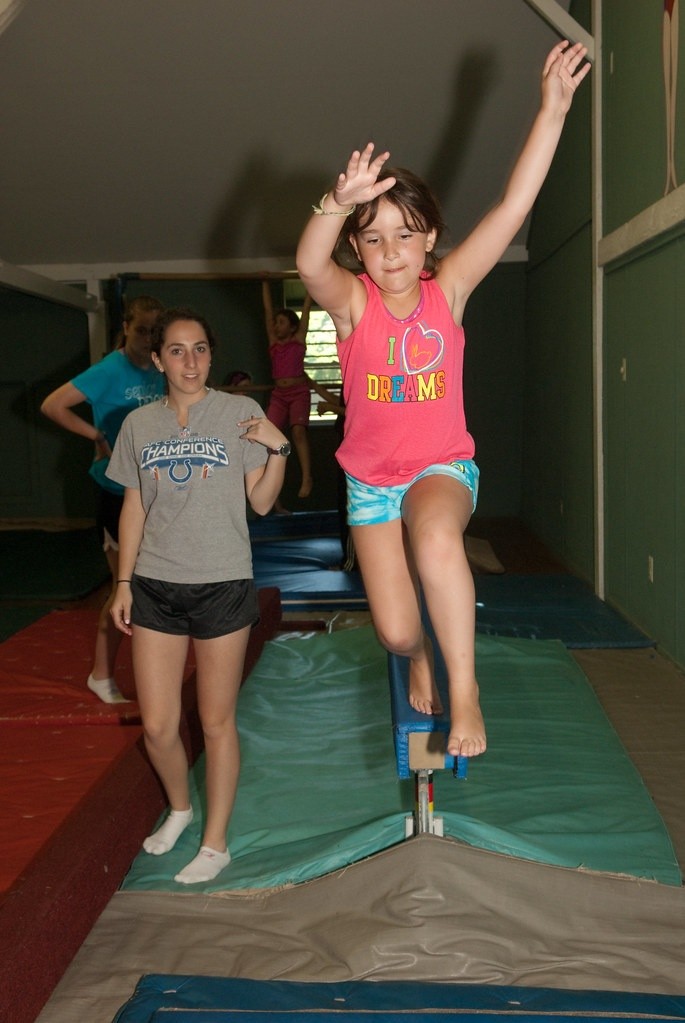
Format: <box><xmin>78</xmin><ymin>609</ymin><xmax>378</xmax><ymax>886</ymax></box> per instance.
<box><xmin>312</xmin><ymin>193</ymin><xmax>357</xmax><ymax>216</ymax></box>
<box><xmin>116</xmin><ymin>580</ymin><xmax>132</xmax><ymax>584</ymax></box>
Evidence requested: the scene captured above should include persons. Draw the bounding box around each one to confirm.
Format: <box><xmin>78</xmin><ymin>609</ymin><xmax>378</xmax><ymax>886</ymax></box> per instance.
<box><xmin>304</xmin><ymin>371</ymin><xmax>358</xmax><ymax>571</ymax></box>
<box><xmin>231</xmin><ymin>373</ymin><xmax>250</xmax><ymax>396</ymax></box>
<box><xmin>259</xmin><ymin>273</ymin><xmax>313</xmax><ymax>498</ymax></box>
<box><xmin>105</xmin><ymin>308</ymin><xmax>290</xmax><ymax>883</ymax></box>
<box><xmin>294</xmin><ymin>38</ymin><xmax>594</xmax><ymax>756</ymax></box>
<box><xmin>39</xmin><ymin>293</ymin><xmax>166</xmax><ymax>704</ymax></box>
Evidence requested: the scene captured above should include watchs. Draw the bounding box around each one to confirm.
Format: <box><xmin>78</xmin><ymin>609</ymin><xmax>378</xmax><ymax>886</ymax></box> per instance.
<box><xmin>267</xmin><ymin>442</ymin><xmax>292</xmax><ymax>456</ymax></box>
